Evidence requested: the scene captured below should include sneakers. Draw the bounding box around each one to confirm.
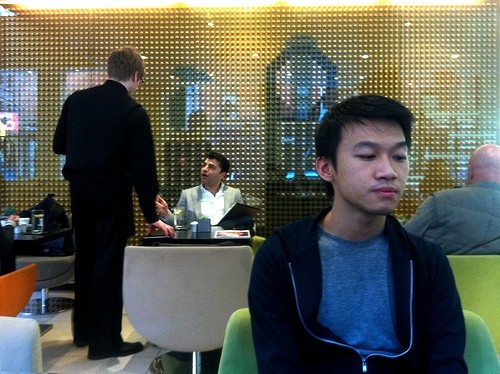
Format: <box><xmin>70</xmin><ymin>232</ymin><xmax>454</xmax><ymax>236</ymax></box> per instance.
<box><xmin>74</xmin><ymin>336</ymin><xmax>89</xmax><ymax>346</ymax></box>
<box><xmin>88</xmin><ymin>342</ymin><xmax>144</xmax><ymax>359</ymax></box>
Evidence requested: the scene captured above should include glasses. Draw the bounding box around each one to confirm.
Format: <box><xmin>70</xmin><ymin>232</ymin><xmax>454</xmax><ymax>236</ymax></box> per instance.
<box><xmin>138</xmin><ymin>73</ymin><xmax>145</xmax><ymax>86</ymax></box>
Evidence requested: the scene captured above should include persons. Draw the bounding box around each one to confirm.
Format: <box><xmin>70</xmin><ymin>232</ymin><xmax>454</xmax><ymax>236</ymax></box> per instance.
<box><xmin>53</xmin><ymin>48</ymin><xmax>176</xmax><ymax>359</ymax></box>
<box><xmin>402</xmin><ymin>144</ymin><xmax>500</xmax><ymax>255</ymax></box>
<box><xmin>0</xmin><ymin>214</ymin><xmax>19</xmax><ymax>276</ymax></box>
<box><xmin>155</xmin><ymin>152</ymin><xmax>254</xmax><ymax>228</ymax></box>
<box><xmin>247</xmin><ymin>94</ymin><xmax>469</xmax><ymax>374</ymax></box>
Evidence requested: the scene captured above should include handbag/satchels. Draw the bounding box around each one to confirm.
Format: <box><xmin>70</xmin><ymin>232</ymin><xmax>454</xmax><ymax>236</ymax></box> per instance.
<box><xmin>19</xmin><ymin>193</ymin><xmax>74</xmax><ymax>256</ymax></box>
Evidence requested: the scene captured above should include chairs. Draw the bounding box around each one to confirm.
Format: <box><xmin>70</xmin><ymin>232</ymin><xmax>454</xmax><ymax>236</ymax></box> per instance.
<box><xmin>15</xmin><ymin>256</ymin><xmax>75</xmax><ymax>314</ymax></box>
<box><xmin>121</xmin><ymin>245</ymin><xmax>254</xmax><ymax>374</ymax></box>
<box><xmin>0</xmin><ymin>263</ymin><xmax>38</xmax><ymax>317</ymax></box>
<box><xmin>447</xmin><ymin>254</ymin><xmax>500</xmax><ymax>361</ymax></box>
<box><xmin>217</xmin><ymin>307</ymin><xmax>500</xmax><ymax>374</ymax></box>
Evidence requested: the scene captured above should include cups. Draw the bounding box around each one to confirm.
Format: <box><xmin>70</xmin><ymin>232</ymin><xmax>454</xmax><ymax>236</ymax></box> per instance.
<box><xmin>174</xmin><ymin>206</ymin><xmax>185</xmax><ymax>228</ymax></box>
<box><xmin>31</xmin><ymin>210</ymin><xmax>43</xmax><ymax>234</ymax></box>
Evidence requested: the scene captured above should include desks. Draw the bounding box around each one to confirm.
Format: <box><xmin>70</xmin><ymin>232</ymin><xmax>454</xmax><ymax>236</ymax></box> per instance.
<box><xmin>142</xmin><ymin>229</ymin><xmax>252</xmax><ymax>246</ymax></box>
<box><xmin>13</xmin><ymin>227</ymin><xmax>74</xmax><ymax>257</ymax></box>
